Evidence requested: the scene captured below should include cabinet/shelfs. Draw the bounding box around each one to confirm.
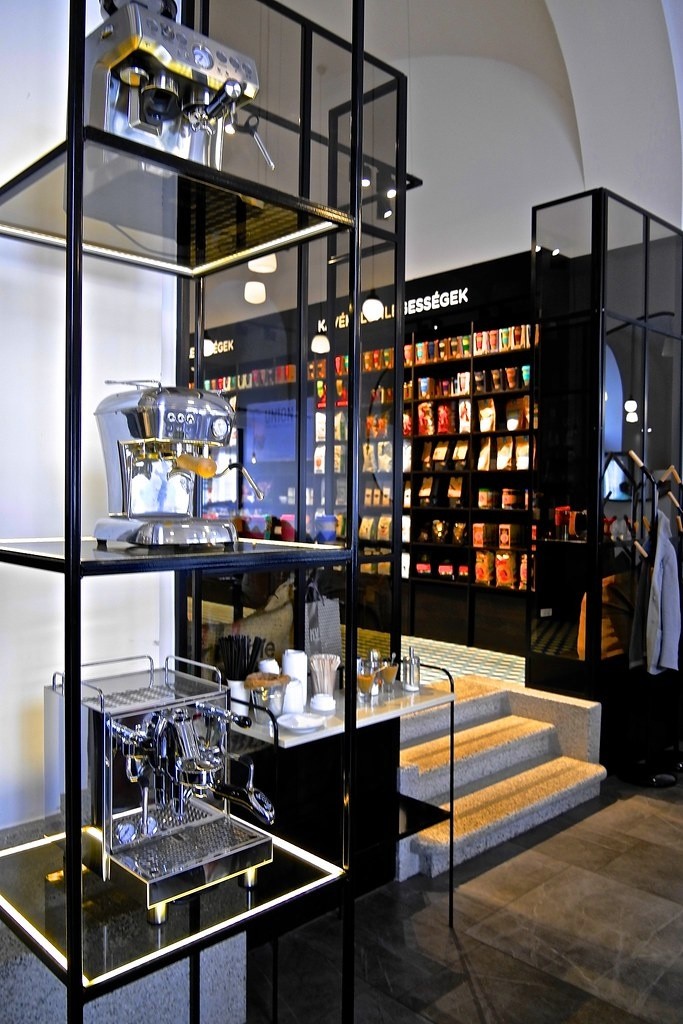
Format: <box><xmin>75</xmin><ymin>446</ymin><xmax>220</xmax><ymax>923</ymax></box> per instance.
<box><xmin>0</xmin><ymin>0</ymin><xmax>682</xmax><ymax>1024</ymax></box>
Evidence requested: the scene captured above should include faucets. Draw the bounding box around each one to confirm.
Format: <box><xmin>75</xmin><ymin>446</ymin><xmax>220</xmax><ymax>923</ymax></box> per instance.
<box><xmin>214</xmin><ymin>760</ymin><xmax>277</xmax><ymax>827</ymax></box>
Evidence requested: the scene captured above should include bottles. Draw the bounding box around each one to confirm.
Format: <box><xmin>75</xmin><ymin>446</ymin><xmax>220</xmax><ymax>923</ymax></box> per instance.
<box><xmin>403</xmin><ymin>647</ymin><xmax>420</xmax><ymax>690</ymax></box>
<box><xmin>283</xmin><ymin>678</ymin><xmax>304</xmax><ymax>713</ymax></box>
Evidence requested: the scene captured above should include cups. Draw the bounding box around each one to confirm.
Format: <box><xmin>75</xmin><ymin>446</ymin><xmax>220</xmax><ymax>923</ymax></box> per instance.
<box><xmin>227</xmin><ymin>680</ymin><xmax>251</xmax><ymax>717</ymax></box>
<box><xmin>380</xmin><ymin>662</ymin><xmax>399</xmax><ymax>695</ymax></box>
<box><xmin>357</xmin><ymin>663</ymin><xmax>374</xmax><ymax>702</ymax></box>
<box><xmin>312</xmin><ymin>670</ymin><xmax>337</xmax><ymax>710</ymax></box>
<box><xmin>283</xmin><ymin>655</ymin><xmax>307</xmax><ymax>705</ymax></box>
<box><xmin>252</xmin><ymin>682</ymin><xmax>287</xmax><ymax>723</ymax></box>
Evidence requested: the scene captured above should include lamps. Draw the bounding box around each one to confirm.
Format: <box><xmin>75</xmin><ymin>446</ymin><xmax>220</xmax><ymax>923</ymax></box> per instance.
<box><xmin>624</xmin><ymin>324</ymin><xmax>640</xmax><ymax>423</ymax></box>
<box><xmin>244</xmin><ymin>0</ymin><xmax>277</xmax><ymax>305</ymax></box>
<box><xmin>359</xmin><ymin>160</ymin><xmax>397</xmax><ymax>221</ymax></box>
<box><xmin>363</xmin><ymin>63</ymin><xmax>384</xmax><ymax>323</ymax></box>
<box><xmin>311</xmin><ymin>65</ymin><xmax>330</xmax><ymax>354</ymax></box>
<box><xmin>203</xmin><ymin>330</ymin><xmax>214</xmax><ymax>358</ymax></box>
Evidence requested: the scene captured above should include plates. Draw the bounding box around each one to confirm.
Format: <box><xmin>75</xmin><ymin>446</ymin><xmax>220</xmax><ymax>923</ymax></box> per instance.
<box><xmin>278</xmin><ymin>712</ymin><xmax>325</xmax><ymax>732</ymax></box>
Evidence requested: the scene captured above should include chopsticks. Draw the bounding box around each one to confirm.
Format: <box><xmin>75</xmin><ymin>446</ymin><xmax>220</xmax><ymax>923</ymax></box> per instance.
<box><xmin>219</xmin><ymin>635</ymin><xmax>266</xmax><ymax>681</ymax></box>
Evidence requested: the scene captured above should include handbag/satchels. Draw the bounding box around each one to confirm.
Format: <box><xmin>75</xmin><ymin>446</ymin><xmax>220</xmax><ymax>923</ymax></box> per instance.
<box><xmin>304</xmin><ymin>584</ymin><xmax>344</xmax><ymax>673</ymax></box>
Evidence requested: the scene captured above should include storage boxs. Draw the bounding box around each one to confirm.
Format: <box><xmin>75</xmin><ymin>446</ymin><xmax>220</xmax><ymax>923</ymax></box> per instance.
<box><xmin>496</xmin><ymin>523</ymin><xmax>523</xmax><ymax>549</ymax></box>
<box><xmin>472</xmin><ymin>523</ymin><xmax>498</xmax><ymax>548</ymax></box>
